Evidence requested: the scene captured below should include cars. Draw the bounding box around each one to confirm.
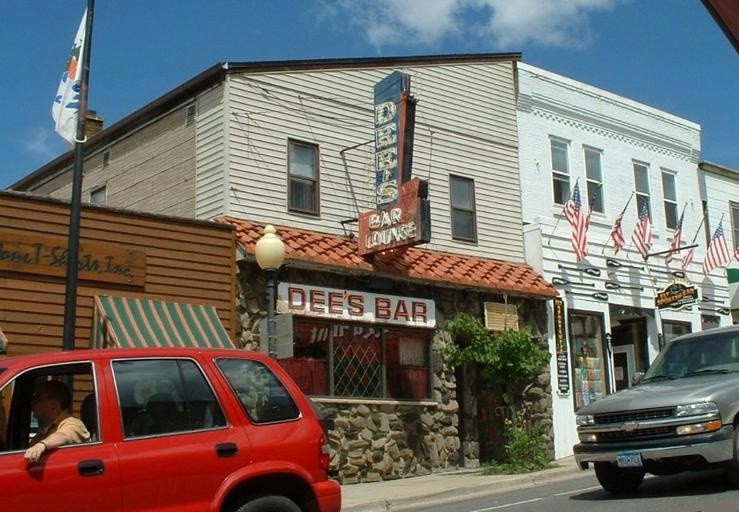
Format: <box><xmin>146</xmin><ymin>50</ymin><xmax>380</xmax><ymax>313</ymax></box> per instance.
<box><xmin>573</xmin><ymin>324</ymin><xmax>739</xmax><ymax>489</ymax></box>
<box><xmin>0</xmin><ymin>348</ymin><xmax>341</xmax><ymax>512</ymax></box>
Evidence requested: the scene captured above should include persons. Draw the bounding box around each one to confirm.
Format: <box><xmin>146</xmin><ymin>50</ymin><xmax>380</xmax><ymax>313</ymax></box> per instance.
<box><xmin>23</xmin><ymin>380</ymin><xmax>92</xmax><ymax>463</ymax></box>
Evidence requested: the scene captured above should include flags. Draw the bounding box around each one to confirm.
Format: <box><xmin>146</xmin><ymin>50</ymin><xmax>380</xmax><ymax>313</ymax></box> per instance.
<box><xmin>702</xmin><ymin>218</ymin><xmax>731</xmax><ymax>276</ymax></box>
<box><xmin>662</xmin><ymin>212</ymin><xmax>685</xmax><ymax>266</ymax></box>
<box><xmin>631</xmin><ymin>204</ymin><xmax>656</xmax><ymax>263</ymax></box>
<box><xmin>730</xmin><ymin>246</ymin><xmax>739</xmax><ymax>266</ymax></box>
<box><xmin>564</xmin><ymin>183</ymin><xmax>589</xmax><ymax>264</ymax></box>
<box><xmin>49</xmin><ymin>5</ymin><xmax>88</xmax><ymax>147</ymax></box>
<box><xmin>610</xmin><ymin>210</ymin><xmax>626</xmax><ymax>255</ymax></box>
<box><xmin>680</xmin><ymin>238</ymin><xmax>696</xmax><ymax>271</ymax></box>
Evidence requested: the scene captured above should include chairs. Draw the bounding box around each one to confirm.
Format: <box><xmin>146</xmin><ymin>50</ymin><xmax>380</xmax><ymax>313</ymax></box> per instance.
<box><xmin>80</xmin><ymin>395</ymin><xmax>98</xmax><ymax>445</ymax></box>
<box><xmin>135</xmin><ymin>393</ymin><xmax>187</xmax><ymax>435</ymax></box>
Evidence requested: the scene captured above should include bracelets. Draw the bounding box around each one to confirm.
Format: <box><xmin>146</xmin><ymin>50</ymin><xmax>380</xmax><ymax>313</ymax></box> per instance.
<box><xmin>37</xmin><ymin>441</ymin><xmax>47</xmax><ymax>451</ymax></box>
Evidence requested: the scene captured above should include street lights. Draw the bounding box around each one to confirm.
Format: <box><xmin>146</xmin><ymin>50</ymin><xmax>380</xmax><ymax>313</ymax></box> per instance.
<box><xmin>255</xmin><ymin>225</ymin><xmax>285</xmax><ymax>361</ymax></box>
